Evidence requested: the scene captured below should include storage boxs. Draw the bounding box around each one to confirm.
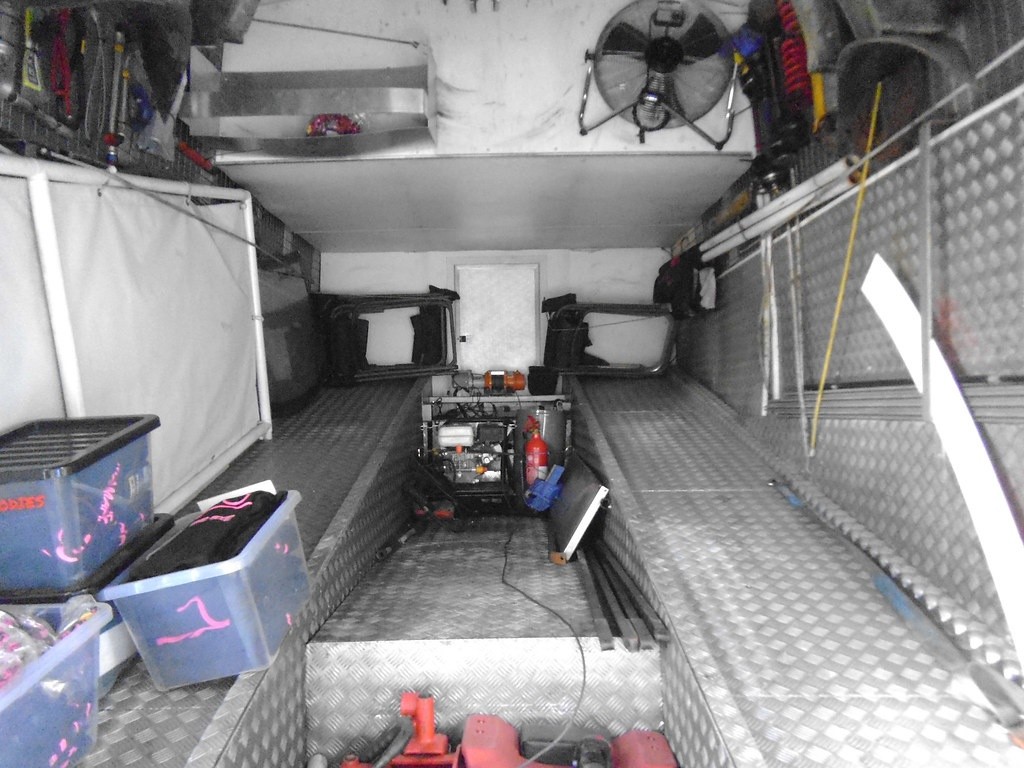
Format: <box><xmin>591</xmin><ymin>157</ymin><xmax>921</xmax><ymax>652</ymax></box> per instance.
<box><xmin>1</xmin><ymin>415</ymin><xmax>163</xmax><ymax>591</ymax></box>
<box><xmin>0</xmin><ymin>602</ymin><xmax>115</xmax><ymax>768</ymax></box>
<box><xmin>1</xmin><ymin>514</ymin><xmax>176</xmax><ymax>701</ymax></box>
<box><xmin>95</xmin><ymin>489</ymin><xmax>315</xmax><ymax>692</ymax></box>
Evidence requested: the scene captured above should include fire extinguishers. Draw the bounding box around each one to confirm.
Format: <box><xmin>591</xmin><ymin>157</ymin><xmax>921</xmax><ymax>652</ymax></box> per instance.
<box><xmin>523</xmin><ymin>417</ymin><xmax>549</xmax><ymax>486</ymax></box>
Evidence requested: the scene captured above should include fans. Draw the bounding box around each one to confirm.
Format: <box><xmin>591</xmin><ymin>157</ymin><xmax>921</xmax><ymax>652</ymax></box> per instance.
<box><xmin>579</xmin><ymin>1</ymin><xmax>740</xmax><ymax>151</ymax></box>
